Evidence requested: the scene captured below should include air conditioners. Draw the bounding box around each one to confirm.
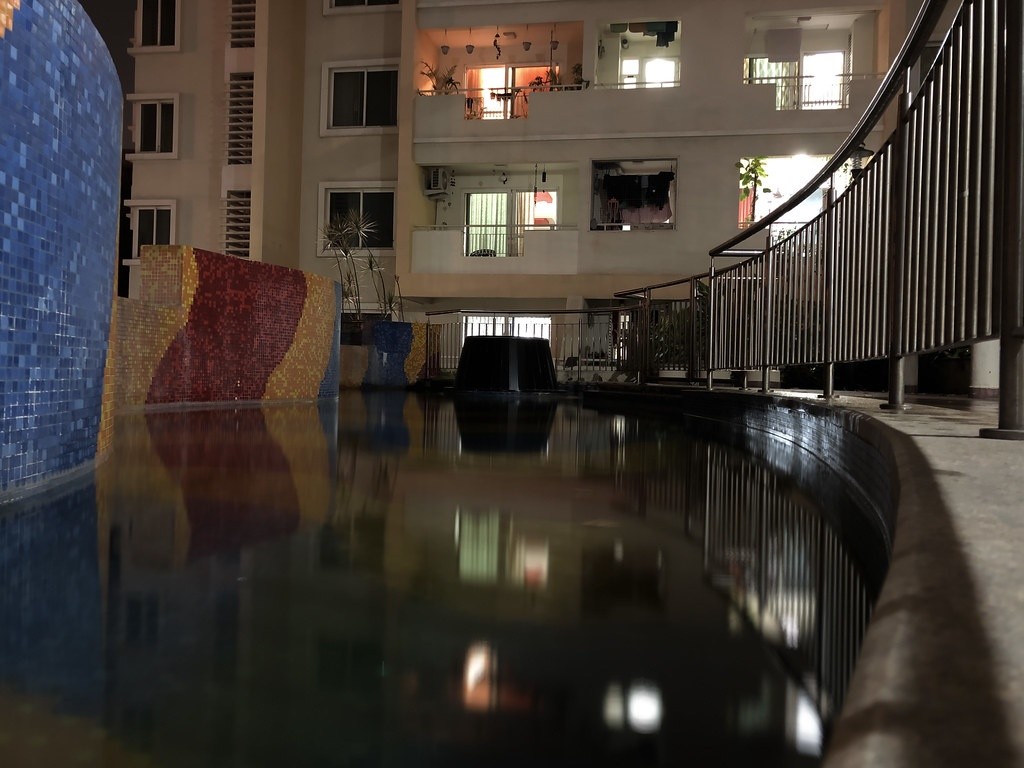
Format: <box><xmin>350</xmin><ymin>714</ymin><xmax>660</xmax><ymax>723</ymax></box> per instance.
<box><xmin>431</xmin><ymin>167</ymin><xmax>450</xmax><ymax>195</ymax></box>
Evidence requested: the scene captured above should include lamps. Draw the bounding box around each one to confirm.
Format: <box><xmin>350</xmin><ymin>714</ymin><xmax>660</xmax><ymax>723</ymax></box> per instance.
<box><xmin>550</xmin><ymin>40</ymin><xmax>559</xmax><ymax>50</ymax></box>
<box><xmin>848</xmin><ymin>142</ymin><xmax>875</xmax><ymax>180</ymax></box>
<box><xmin>502</xmin><ymin>32</ymin><xmax>516</xmax><ymax>40</ymax></box>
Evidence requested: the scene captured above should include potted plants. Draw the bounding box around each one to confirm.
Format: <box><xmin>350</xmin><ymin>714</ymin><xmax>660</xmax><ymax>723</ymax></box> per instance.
<box><xmin>649</xmin><ymin>306</ymin><xmax>706</xmax><ymax>387</ymax></box>
<box><xmin>695</xmin><ymin>272</ymin><xmax>766</xmax><ymax>387</ymax></box>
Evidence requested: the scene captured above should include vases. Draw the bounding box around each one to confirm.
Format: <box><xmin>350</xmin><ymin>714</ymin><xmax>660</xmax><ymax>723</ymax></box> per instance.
<box><xmin>523</xmin><ymin>41</ymin><xmax>532</xmax><ymax>52</ymax></box>
<box><xmin>441</xmin><ymin>45</ymin><xmax>450</xmax><ymax>55</ymax></box>
<box><xmin>466</xmin><ymin>45</ymin><xmax>475</xmax><ymax>55</ymax></box>
